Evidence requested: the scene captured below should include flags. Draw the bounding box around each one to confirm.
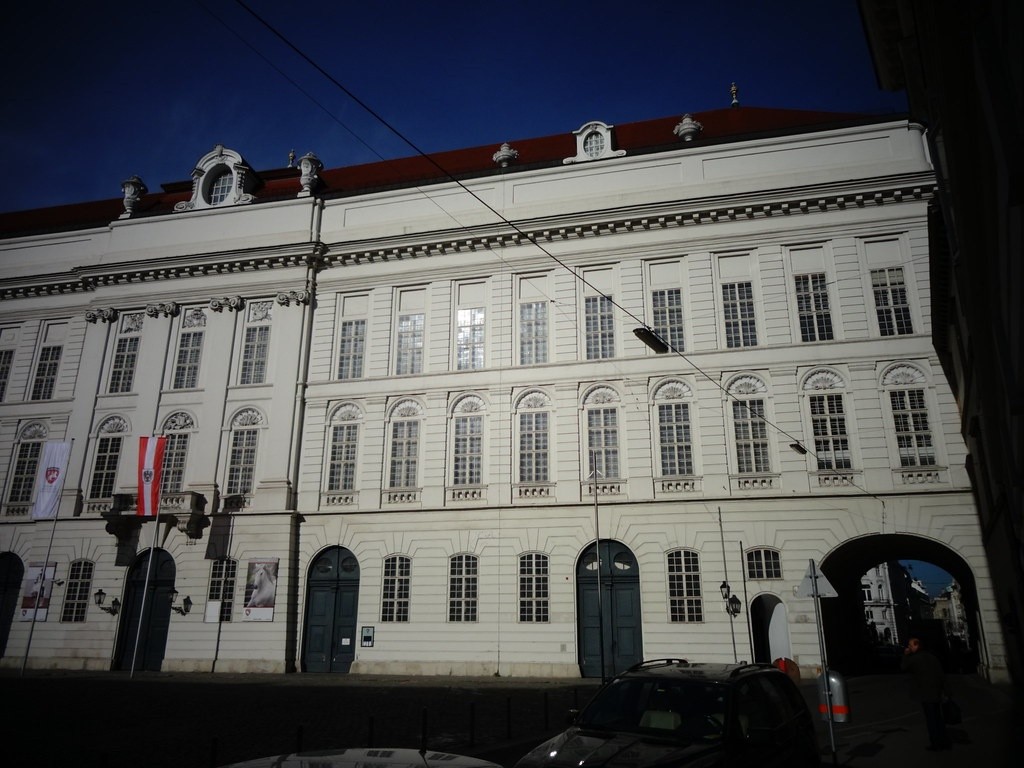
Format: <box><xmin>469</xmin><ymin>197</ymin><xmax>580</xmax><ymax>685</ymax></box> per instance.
<box><xmin>31</xmin><ymin>441</ymin><xmax>71</xmax><ymax>518</ymax></box>
<box><xmin>136</xmin><ymin>437</ymin><xmax>166</xmax><ymax>516</ymax></box>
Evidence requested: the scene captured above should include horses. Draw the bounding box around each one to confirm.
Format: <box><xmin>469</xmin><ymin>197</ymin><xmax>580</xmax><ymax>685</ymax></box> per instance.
<box><xmin>247</xmin><ymin>562</ymin><xmax>276</xmax><ymax>608</ymax></box>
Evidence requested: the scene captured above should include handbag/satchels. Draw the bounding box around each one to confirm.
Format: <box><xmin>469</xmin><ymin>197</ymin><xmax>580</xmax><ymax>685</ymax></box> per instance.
<box><xmin>941</xmin><ymin>700</ymin><xmax>962</xmax><ymax>725</ymax></box>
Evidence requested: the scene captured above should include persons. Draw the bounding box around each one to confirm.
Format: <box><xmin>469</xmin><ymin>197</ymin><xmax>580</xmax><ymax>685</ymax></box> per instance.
<box><xmin>900</xmin><ymin>638</ymin><xmax>951</xmax><ymax>752</ymax></box>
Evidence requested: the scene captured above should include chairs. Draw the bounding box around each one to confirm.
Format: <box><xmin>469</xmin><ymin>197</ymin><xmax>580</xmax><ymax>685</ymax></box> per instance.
<box><xmin>640</xmin><ymin>689</ymin><xmax>683</xmax><ymax>730</ymax></box>
<box><xmin>709</xmin><ymin>685</ymin><xmax>751</xmax><ymax>737</ymax></box>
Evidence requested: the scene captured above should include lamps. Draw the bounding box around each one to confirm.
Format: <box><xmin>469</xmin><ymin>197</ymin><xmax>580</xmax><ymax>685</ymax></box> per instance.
<box><xmin>632</xmin><ymin>328</ymin><xmax>668</xmax><ymax>354</ymax></box>
<box><xmin>109</xmin><ymin>598</ymin><xmax>122</xmax><ymax>616</ymax></box>
<box><xmin>94</xmin><ymin>589</ymin><xmax>112</xmax><ymax>613</ymax></box>
<box><xmin>180</xmin><ymin>596</ymin><xmax>192</xmax><ymax>616</ymax></box>
<box><xmin>720</xmin><ymin>581</ymin><xmax>732</xmax><ymax>614</ymax></box>
<box><xmin>789</xmin><ymin>444</ymin><xmax>806</xmax><ymax>455</ymax></box>
<box><xmin>728</xmin><ymin>594</ymin><xmax>741</xmax><ymax>617</ymax></box>
<box><xmin>166</xmin><ymin>587</ymin><xmax>183</xmax><ymax>614</ymax></box>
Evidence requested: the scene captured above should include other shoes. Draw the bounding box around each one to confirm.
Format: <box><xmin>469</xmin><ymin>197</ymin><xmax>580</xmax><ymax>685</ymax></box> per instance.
<box><xmin>925</xmin><ymin>744</ymin><xmax>942</xmax><ymax>752</ymax></box>
<box><xmin>940</xmin><ymin>743</ymin><xmax>952</xmax><ymax>751</ymax></box>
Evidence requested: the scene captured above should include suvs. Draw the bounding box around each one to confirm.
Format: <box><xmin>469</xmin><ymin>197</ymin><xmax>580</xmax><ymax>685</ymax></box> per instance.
<box><xmin>516</xmin><ymin>657</ymin><xmax>819</xmax><ymax>768</ymax></box>
